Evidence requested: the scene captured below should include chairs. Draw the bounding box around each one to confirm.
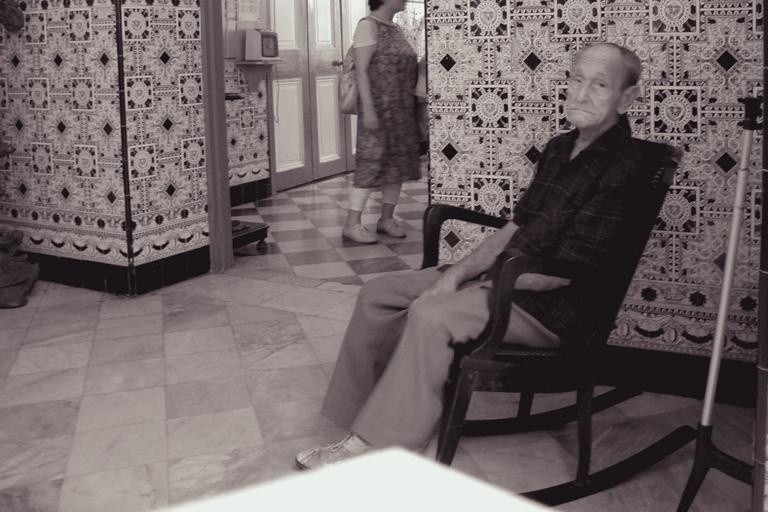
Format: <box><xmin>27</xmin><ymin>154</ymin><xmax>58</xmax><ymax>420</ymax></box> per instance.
<box><xmin>423</xmin><ymin>139</ymin><xmax>699</xmax><ymax>506</ymax></box>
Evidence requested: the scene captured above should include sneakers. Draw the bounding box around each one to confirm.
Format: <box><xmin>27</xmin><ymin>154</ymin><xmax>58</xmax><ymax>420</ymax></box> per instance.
<box><xmin>377</xmin><ymin>218</ymin><xmax>406</xmax><ymax>238</ymax></box>
<box><xmin>342</xmin><ymin>223</ymin><xmax>378</xmax><ymax>244</ymax></box>
<box><xmin>296</xmin><ymin>433</ymin><xmax>369</xmax><ymax>470</ymax></box>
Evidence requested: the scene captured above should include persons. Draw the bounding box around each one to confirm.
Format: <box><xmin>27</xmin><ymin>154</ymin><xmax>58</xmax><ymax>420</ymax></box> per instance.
<box><xmin>293</xmin><ymin>43</ymin><xmax>648</xmax><ymax>473</ymax></box>
<box><xmin>340</xmin><ymin>0</ymin><xmax>419</xmax><ymax>245</ymax></box>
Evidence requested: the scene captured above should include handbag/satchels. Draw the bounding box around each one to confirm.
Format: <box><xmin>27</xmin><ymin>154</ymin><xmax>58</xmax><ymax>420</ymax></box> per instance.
<box><xmin>338</xmin><ymin>47</ymin><xmax>360</xmax><ymax>114</ymax></box>
